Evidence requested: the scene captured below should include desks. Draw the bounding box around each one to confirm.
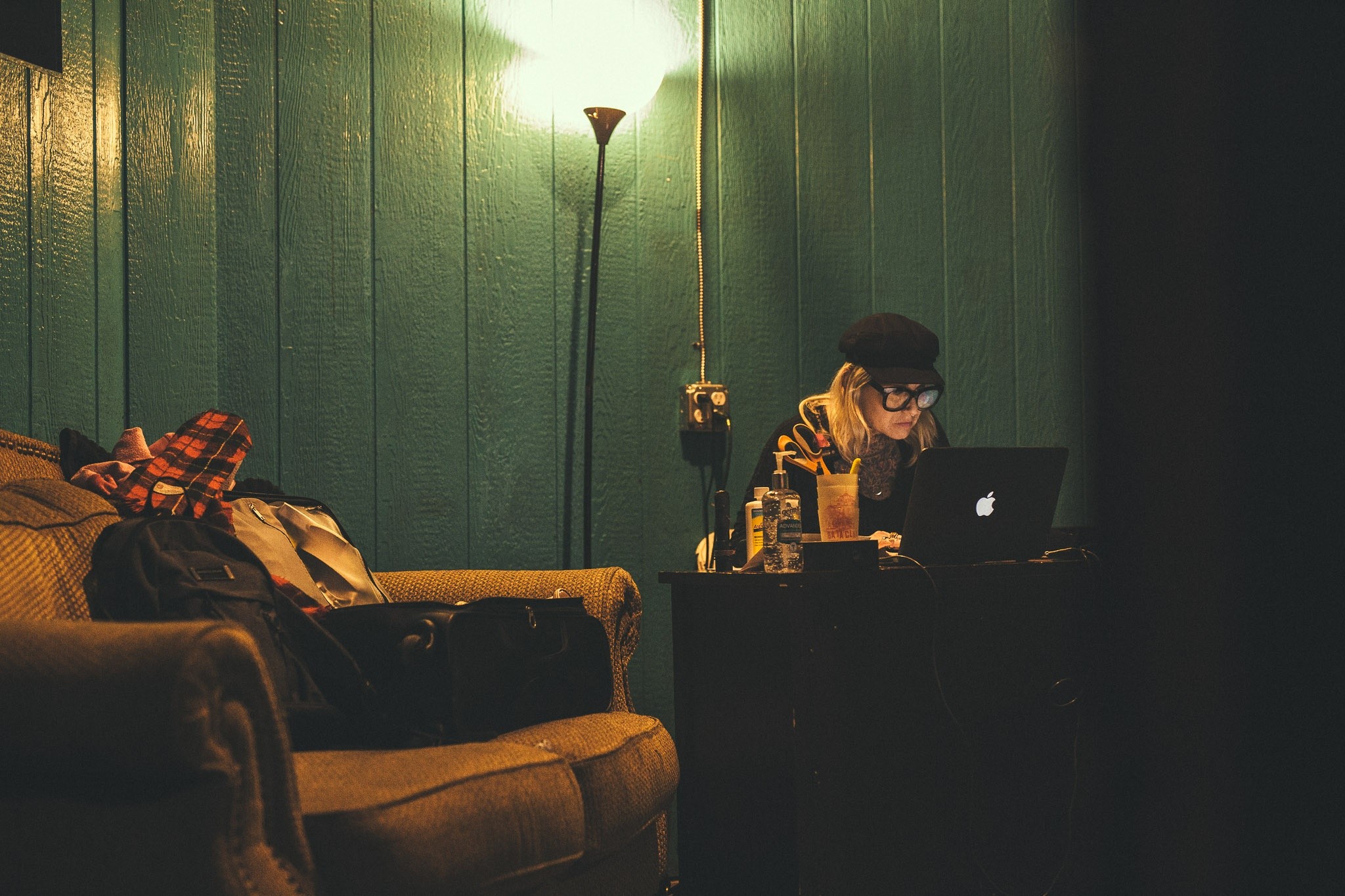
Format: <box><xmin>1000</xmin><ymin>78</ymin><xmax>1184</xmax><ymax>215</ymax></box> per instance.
<box><xmin>657</xmin><ymin>560</ymin><xmax>1098</xmax><ymax>896</ymax></box>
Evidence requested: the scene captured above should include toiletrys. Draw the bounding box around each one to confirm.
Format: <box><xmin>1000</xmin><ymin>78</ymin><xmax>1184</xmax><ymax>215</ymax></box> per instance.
<box><xmin>761</xmin><ymin>451</ymin><xmax>804</xmax><ymax>574</ymax></box>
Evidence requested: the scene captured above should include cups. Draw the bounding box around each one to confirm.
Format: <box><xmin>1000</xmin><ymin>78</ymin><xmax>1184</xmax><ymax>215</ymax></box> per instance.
<box><xmin>816</xmin><ymin>474</ymin><xmax>859</xmax><ymax>542</ymax></box>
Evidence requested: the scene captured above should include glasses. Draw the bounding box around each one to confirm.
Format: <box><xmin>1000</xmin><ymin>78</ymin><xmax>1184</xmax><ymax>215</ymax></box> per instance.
<box><xmin>868</xmin><ymin>380</ymin><xmax>942</xmax><ymax>412</ymax></box>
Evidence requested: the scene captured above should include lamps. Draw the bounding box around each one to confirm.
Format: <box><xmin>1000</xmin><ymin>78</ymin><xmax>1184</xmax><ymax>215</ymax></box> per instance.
<box><xmin>583</xmin><ymin>6</ymin><xmax>681</xmax><ymax>568</ymax></box>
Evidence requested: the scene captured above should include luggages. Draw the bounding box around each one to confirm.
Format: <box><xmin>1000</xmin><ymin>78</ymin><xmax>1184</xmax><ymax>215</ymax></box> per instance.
<box><xmin>312</xmin><ymin>597</ymin><xmax>615</xmax><ymax>749</ymax></box>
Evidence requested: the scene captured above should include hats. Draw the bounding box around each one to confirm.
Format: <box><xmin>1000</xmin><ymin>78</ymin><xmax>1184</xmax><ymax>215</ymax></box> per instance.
<box><xmin>839</xmin><ymin>313</ymin><xmax>946</xmax><ymax>385</ymax></box>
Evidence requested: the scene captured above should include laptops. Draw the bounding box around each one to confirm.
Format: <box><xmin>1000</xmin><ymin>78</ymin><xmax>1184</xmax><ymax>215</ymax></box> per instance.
<box><xmin>880</xmin><ymin>446</ymin><xmax>1071</xmax><ymax>567</ymax></box>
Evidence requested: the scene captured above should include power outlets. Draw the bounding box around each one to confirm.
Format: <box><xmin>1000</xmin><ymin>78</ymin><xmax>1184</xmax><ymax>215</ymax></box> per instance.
<box><xmin>678</xmin><ymin>383</ymin><xmax>730</xmax><ymax>432</ymax></box>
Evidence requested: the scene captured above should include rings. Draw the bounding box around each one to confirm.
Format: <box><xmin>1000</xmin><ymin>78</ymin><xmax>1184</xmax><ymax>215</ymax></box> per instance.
<box><xmin>882</xmin><ymin>536</ymin><xmax>887</xmax><ymax>540</ymax></box>
<box><xmin>890</xmin><ymin>532</ymin><xmax>898</xmax><ymax>538</ymax></box>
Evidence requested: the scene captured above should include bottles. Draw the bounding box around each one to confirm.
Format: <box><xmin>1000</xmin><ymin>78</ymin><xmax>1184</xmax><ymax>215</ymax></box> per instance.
<box><xmin>746</xmin><ymin>488</ymin><xmax>770</xmax><ymax>563</ymax></box>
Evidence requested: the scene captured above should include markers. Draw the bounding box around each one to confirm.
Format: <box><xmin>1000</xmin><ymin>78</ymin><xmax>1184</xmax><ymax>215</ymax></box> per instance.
<box><xmin>851</xmin><ymin>458</ymin><xmax>861</xmax><ymax>474</ymax></box>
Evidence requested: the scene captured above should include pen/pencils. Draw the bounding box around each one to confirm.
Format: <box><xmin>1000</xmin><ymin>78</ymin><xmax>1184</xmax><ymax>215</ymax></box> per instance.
<box><xmin>816</xmin><ymin>461</ymin><xmax>824</xmax><ymax>475</ymax></box>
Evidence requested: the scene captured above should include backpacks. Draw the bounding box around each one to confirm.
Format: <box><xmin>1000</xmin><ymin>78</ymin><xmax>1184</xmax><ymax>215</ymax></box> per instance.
<box><xmin>83</xmin><ymin>476</ymin><xmax>394</xmax><ymax>749</ymax></box>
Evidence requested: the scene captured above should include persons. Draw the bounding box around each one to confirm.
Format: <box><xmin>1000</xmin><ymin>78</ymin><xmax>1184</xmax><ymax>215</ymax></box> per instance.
<box><xmin>729</xmin><ymin>313</ymin><xmax>950</xmax><ymax>571</ymax></box>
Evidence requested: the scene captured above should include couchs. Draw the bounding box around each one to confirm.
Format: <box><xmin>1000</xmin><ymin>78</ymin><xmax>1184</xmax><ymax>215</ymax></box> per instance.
<box><xmin>0</xmin><ymin>428</ymin><xmax>681</xmax><ymax>896</ymax></box>
